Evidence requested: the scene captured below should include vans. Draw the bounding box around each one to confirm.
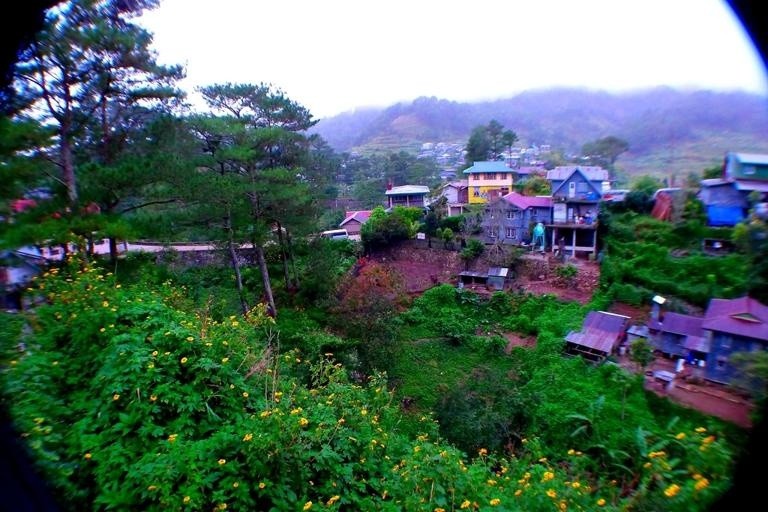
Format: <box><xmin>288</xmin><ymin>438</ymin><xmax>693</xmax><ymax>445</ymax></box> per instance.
<box><xmin>320</xmin><ymin>229</ymin><xmax>348</xmax><ymax>240</ymax></box>
